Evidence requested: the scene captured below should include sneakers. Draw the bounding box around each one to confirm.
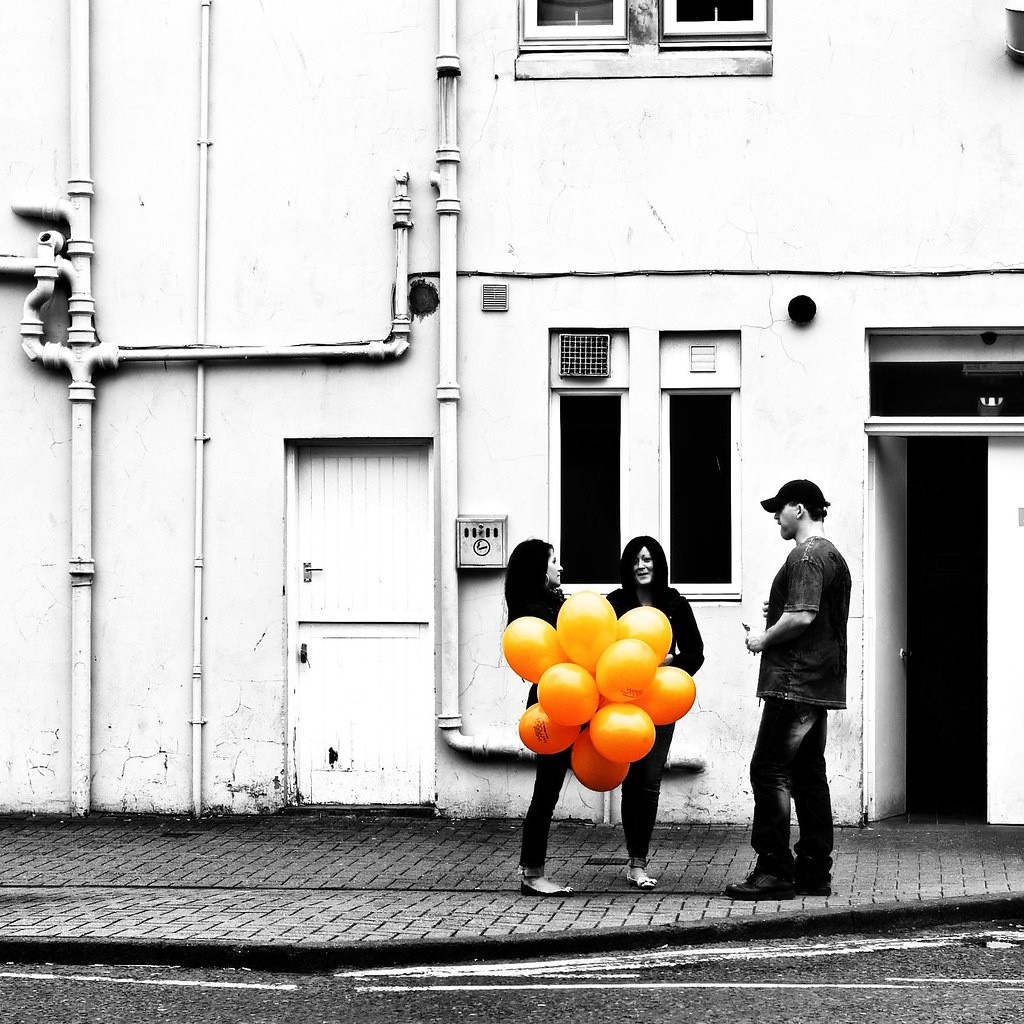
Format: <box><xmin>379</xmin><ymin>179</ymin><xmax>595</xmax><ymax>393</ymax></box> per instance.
<box><xmin>724</xmin><ymin>869</ymin><xmax>796</xmax><ymax>900</ymax></box>
<box><xmin>791</xmin><ymin>879</ymin><xmax>832</xmax><ymax>897</ymax></box>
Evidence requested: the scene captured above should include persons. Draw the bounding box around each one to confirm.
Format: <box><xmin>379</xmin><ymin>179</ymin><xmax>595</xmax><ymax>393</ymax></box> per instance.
<box><xmin>505</xmin><ymin>539</ymin><xmax>588</xmax><ymax>897</ymax></box>
<box><xmin>725</xmin><ymin>479</ymin><xmax>852</xmax><ymax>900</ymax></box>
<box><xmin>606</xmin><ymin>536</ymin><xmax>705</xmax><ymax>888</ymax></box>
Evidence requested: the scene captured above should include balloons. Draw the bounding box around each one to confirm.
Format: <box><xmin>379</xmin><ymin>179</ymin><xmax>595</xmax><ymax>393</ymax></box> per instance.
<box><xmin>518</xmin><ymin>702</ymin><xmax>581</xmax><ymax>754</ymax></box>
<box><xmin>556</xmin><ymin>590</ymin><xmax>618</xmax><ymax>672</ymax></box>
<box><xmin>572</xmin><ymin>727</ymin><xmax>630</xmax><ymax>791</ymax></box>
<box><xmin>537</xmin><ymin>663</ymin><xmax>599</xmax><ymax>726</ymax></box>
<box><xmin>590</xmin><ymin>702</ymin><xmax>655</xmax><ymax>763</ymax></box>
<box><xmin>634</xmin><ymin>667</ymin><xmax>695</xmax><ymax>725</ymax></box>
<box><xmin>617</xmin><ymin>606</ymin><xmax>672</xmax><ymax>666</ymax></box>
<box><xmin>597</xmin><ymin>638</ymin><xmax>657</xmax><ymax>702</ymax></box>
<box><xmin>502</xmin><ymin>616</ymin><xmax>565</xmax><ymax>683</ymax></box>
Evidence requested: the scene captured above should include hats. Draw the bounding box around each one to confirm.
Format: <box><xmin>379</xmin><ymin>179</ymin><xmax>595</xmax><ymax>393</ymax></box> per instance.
<box><xmin>760</xmin><ymin>479</ymin><xmax>826</xmax><ymax>513</ymax></box>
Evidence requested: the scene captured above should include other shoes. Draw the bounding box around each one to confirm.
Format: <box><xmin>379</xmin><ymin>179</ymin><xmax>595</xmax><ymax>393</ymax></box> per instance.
<box><xmin>520</xmin><ymin>880</ymin><xmax>575</xmax><ymax>897</ymax></box>
<box><xmin>626</xmin><ymin>868</ymin><xmax>659</xmax><ymax>889</ymax></box>
